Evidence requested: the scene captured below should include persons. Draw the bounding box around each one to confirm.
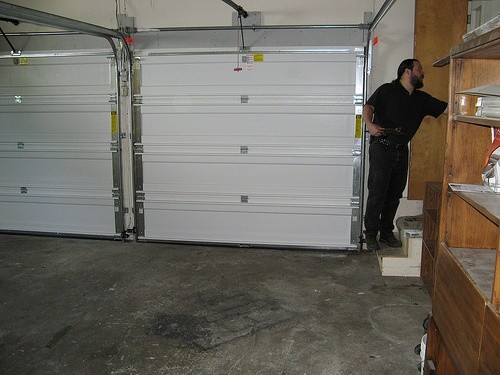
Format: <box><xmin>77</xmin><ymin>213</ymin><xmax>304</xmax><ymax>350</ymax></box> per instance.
<box><xmin>363</xmin><ymin>58</ymin><xmax>448</xmax><ymax>251</ymax></box>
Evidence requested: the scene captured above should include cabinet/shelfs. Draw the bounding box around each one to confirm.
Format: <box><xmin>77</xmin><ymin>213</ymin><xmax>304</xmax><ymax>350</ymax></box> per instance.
<box><xmin>423</xmin><ymin>27</ymin><xmax>500</xmax><ymax>375</ymax></box>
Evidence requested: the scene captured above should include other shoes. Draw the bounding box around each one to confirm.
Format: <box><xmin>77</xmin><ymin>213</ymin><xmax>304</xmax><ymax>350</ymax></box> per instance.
<box><xmin>365</xmin><ymin>233</ymin><xmax>379</xmax><ymax>250</ymax></box>
<box><xmin>379</xmin><ymin>232</ymin><xmax>401</xmax><ymax>247</ymax></box>
